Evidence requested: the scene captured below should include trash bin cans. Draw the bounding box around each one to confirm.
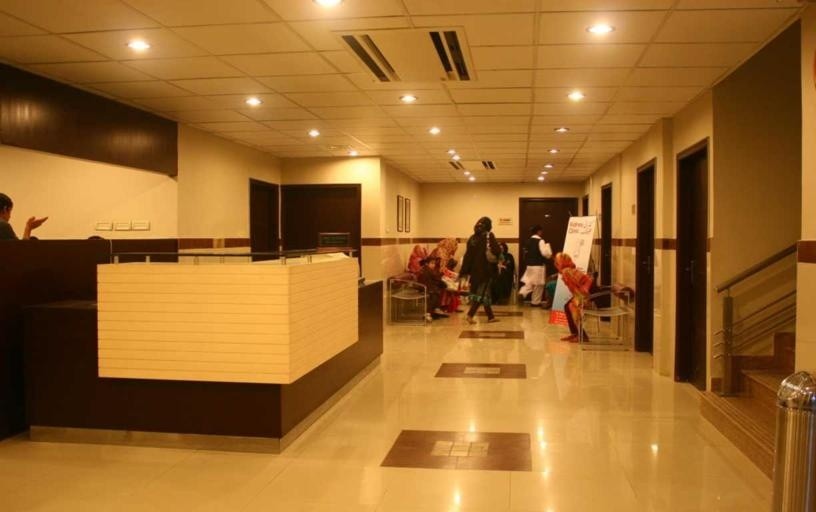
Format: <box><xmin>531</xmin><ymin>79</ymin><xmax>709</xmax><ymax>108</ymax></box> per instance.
<box><xmin>771</xmin><ymin>370</ymin><xmax>816</xmax><ymax>512</ymax></box>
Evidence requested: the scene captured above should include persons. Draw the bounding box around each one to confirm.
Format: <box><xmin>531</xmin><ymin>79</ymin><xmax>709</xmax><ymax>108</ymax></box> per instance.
<box><xmin>554</xmin><ymin>251</ymin><xmax>599</xmax><ymax>343</ymax></box>
<box><xmin>0</xmin><ymin>191</ymin><xmax>50</xmax><ymax>242</ymax></box>
<box><xmin>493</xmin><ymin>241</ymin><xmax>514</xmax><ymax>304</ymax></box>
<box><xmin>462</xmin><ymin>215</ymin><xmax>502</xmax><ymax>325</ymax></box>
<box><xmin>406</xmin><ymin>235</ymin><xmax>464</xmax><ymax>318</ymax></box>
<box><xmin>514</xmin><ymin>225</ymin><xmax>552</xmax><ymax>307</ymax></box>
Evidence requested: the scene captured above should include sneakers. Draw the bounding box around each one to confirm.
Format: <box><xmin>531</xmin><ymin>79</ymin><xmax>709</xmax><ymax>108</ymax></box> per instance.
<box><xmin>423</xmin><ymin>305</ymin><xmax>500</xmax><ymax>324</ymax></box>
<box><xmin>518</xmin><ymin>294</ymin><xmax>543</xmax><ymax>307</ymax></box>
<box><xmin>560</xmin><ymin>334</ymin><xmax>589</xmax><ymax>343</ymax></box>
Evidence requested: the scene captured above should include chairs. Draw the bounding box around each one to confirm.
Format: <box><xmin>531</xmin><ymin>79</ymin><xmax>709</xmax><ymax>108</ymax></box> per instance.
<box><xmin>389</xmin><ymin>273</ymin><xmax>433</xmax><ymax>326</ymax></box>
<box><xmin>579</xmin><ymin>286</ymin><xmax>634</xmax><ymax>352</ymax></box>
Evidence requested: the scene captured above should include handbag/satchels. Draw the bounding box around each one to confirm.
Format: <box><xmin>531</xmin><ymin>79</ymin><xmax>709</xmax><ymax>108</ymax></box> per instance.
<box><xmin>485</xmin><ymin>231</ymin><xmax>504</xmax><ymax>264</ymax></box>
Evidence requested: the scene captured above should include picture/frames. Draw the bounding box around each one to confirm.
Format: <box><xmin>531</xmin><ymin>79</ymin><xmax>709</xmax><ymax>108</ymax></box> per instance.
<box><xmin>398</xmin><ymin>196</ymin><xmax>410</xmax><ymax>232</ymax></box>
